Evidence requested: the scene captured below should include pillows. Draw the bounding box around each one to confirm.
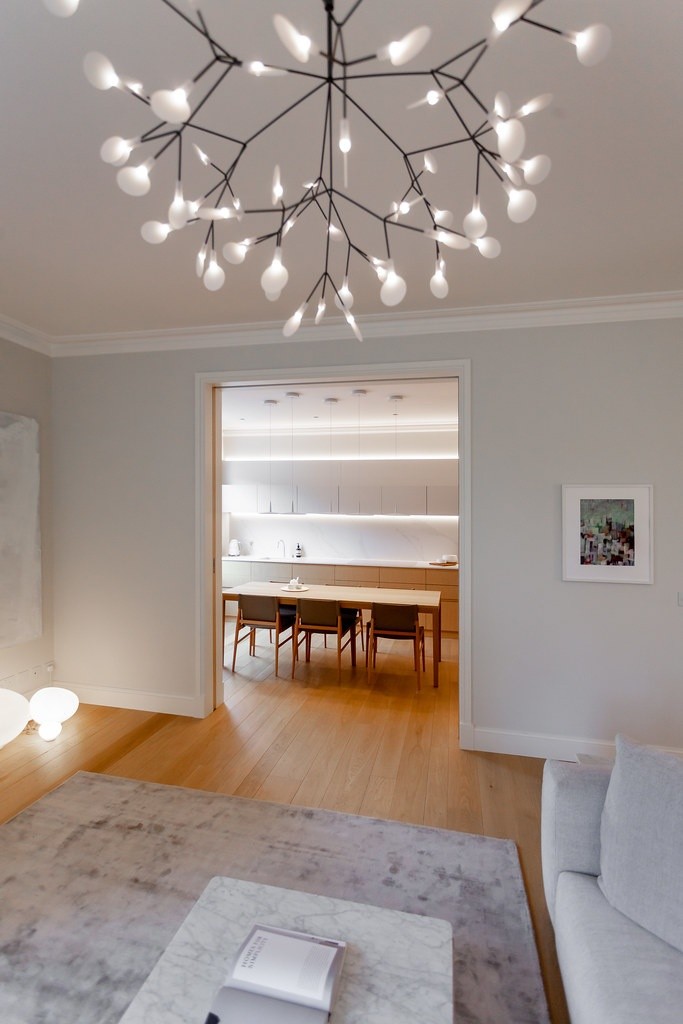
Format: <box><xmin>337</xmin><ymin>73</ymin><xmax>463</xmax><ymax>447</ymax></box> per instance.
<box><xmin>598</xmin><ymin>733</ymin><xmax>683</xmax><ymax>952</ymax></box>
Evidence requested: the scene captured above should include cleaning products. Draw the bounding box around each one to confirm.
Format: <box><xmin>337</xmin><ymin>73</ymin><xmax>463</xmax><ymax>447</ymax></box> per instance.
<box><xmin>295</xmin><ymin>543</ymin><xmax>301</xmax><ymax>558</ymax></box>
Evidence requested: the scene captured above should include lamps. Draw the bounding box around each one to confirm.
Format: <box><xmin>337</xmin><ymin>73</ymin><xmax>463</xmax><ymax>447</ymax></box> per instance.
<box><xmin>40</xmin><ymin>0</ymin><xmax>616</xmax><ymax>344</ymax></box>
<box><xmin>258</xmin><ymin>389</ymin><xmax>435</xmax><ymax>517</ymax></box>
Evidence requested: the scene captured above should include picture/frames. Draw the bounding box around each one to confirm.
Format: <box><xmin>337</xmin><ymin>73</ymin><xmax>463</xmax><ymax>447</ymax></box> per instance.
<box><xmin>561</xmin><ymin>484</ymin><xmax>655</xmax><ymax>584</ymax></box>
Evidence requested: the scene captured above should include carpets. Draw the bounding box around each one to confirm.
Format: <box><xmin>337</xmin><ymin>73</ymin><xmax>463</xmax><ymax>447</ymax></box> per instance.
<box><xmin>0</xmin><ymin>771</ymin><xmax>549</xmax><ymax>1024</ymax></box>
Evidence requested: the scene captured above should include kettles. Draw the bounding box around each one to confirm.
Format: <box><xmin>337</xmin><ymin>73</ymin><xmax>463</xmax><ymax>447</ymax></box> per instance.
<box><xmin>228</xmin><ymin>539</ymin><xmax>241</xmax><ymax>556</ymax></box>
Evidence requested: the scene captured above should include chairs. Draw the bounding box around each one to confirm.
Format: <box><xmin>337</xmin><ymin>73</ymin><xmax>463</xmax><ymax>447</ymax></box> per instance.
<box><xmin>232</xmin><ymin>582</ymin><xmax>424</xmax><ymax>690</ymax></box>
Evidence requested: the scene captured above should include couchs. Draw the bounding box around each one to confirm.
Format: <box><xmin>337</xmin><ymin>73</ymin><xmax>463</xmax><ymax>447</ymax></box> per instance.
<box><xmin>541</xmin><ymin>732</ymin><xmax>683</xmax><ymax>1024</ymax></box>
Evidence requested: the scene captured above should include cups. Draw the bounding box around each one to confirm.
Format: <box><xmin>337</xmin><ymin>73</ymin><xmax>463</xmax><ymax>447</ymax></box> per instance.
<box><xmin>295</xmin><ymin>584</ymin><xmax>302</xmax><ymax>589</ymax></box>
<box><xmin>288</xmin><ymin>584</ymin><xmax>293</xmax><ymax>589</ymax></box>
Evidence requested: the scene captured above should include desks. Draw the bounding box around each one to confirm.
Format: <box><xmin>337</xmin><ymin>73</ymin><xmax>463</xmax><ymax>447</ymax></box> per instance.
<box><xmin>118</xmin><ymin>876</ymin><xmax>455</xmax><ymax>1023</ymax></box>
<box><xmin>223</xmin><ymin>584</ymin><xmax>442</xmax><ymax>688</ymax></box>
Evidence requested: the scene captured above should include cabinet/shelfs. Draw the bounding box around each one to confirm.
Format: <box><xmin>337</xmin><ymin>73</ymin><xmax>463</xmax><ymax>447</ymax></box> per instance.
<box><xmin>222</xmin><ymin>559</ymin><xmax>459</xmax><ymax>640</ymax></box>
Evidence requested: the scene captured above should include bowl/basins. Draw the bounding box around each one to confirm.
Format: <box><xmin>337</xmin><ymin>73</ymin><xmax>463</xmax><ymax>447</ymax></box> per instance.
<box><xmin>443</xmin><ymin>554</ymin><xmax>458</xmax><ymax>562</ymax></box>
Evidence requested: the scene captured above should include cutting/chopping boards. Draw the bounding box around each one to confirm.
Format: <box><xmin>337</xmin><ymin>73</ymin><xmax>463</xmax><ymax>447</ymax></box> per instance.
<box><xmin>428</xmin><ymin>561</ymin><xmax>457</xmax><ymax>566</ymax></box>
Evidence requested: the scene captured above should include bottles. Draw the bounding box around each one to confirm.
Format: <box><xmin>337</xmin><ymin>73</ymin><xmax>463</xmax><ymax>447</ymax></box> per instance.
<box><xmin>290</xmin><ymin>577</ymin><xmax>298</xmax><ymax>588</ymax></box>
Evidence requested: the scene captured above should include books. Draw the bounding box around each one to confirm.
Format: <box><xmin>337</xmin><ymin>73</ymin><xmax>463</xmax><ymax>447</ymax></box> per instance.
<box><xmin>204</xmin><ymin>923</ymin><xmax>347</xmax><ymax>1024</ymax></box>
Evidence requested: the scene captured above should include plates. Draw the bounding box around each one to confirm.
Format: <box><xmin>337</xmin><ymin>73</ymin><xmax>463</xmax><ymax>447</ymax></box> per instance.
<box><xmin>280</xmin><ymin>587</ymin><xmax>308</xmax><ymax>591</ymax></box>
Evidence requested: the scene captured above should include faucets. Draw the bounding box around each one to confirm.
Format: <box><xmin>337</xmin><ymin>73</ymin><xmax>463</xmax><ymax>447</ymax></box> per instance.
<box><xmin>276</xmin><ymin>539</ymin><xmax>286</xmax><ymax>557</ymax></box>
